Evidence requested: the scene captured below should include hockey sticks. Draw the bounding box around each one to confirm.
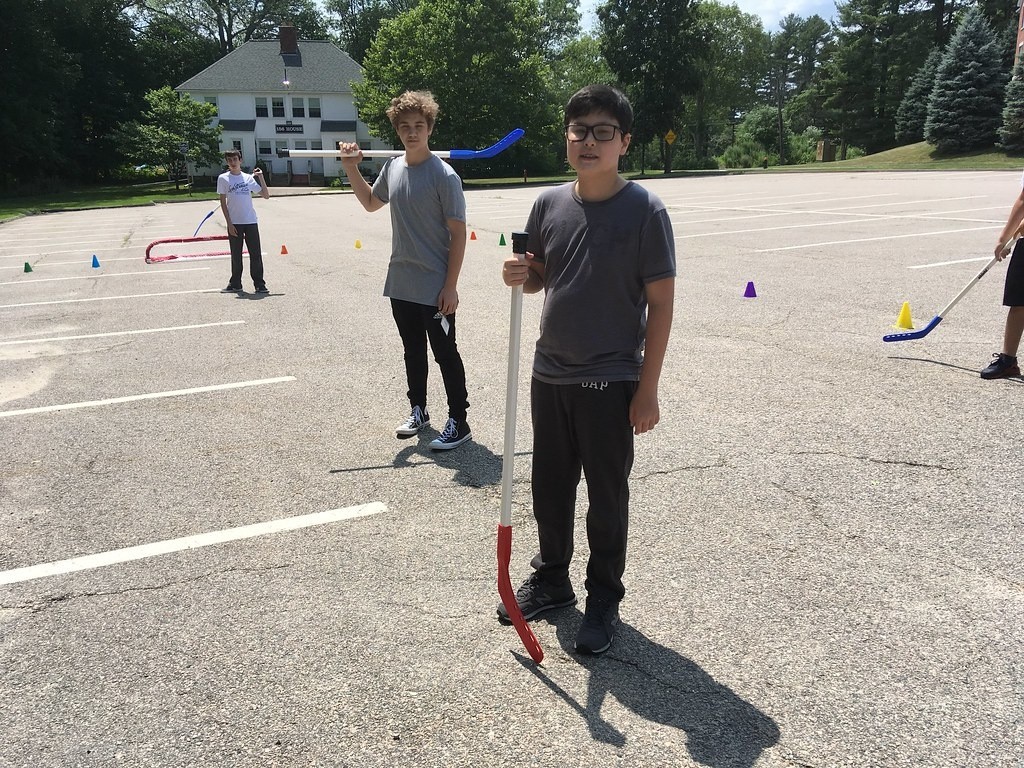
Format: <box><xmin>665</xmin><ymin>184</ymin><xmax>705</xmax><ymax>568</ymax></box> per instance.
<box><xmin>883</xmin><ymin>230</ymin><xmax>1021</xmax><ymax>342</ymax></box>
<box><xmin>277</xmin><ymin>128</ymin><xmax>526</xmax><ymax>159</ymax></box>
<box><xmin>193</xmin><ymin>169</ymin><xmax>260</xmax><ymax>238</ymax></box>
<box><xmin>496</xmin><ymin>233</ymin><xmax>544</xmax><ymax>665</ymax></box>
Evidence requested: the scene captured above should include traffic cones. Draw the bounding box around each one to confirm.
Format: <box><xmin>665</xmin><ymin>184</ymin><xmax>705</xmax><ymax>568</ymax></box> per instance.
<box><xmin>91</xmin><ymin>254</ymin><xmax>100</xmax><ymax>267</ymax></box>
<box><xmin>24</xmin><ymin>262</ymin><xmax>32</xmax><ymax>272</ymax></box>
<box><xmin>469</xmin><ymin>231</ymin><xmax>476</xmax><ymax>240</ymax></box>
<box><xmin>744</xmin><ymin>281</ymin><xmax>758</xmax><ymax>297</ymax></box>
<box><xmin>355</xmin><ymin>238</ymin><xmax>361</xmax><ymax>248</ymax></box>
<box><xmin>280</xmin><ymin>245</ymin><xmax>288</xmax><ymax>254</ymax></box>
<box><xmin>895</xmin><ymin>301</ymin><xmax>915</xmax><ymax>330</ymax></box>
<box><xmin>499</xmin><ymin>233</ymin><xmax>507</xmax><ymax>245</ymax></box>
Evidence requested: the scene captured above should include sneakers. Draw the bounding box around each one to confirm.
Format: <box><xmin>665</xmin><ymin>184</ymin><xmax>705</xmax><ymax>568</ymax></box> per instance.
<box><xmin>220</xmin><ymin>284</ymin><xmax>243</xmax><ymax>293</ymax></box>
<box><xmin>981</xmin><ymin>353</ymin><xmax>1021</xmax><ymax>379</ymax></box>
<box><xmin>396</xmin><ymin>405</ymin><xmax>430</xmax><ymax>435</ymax></box>
<box><xmin>497</xmin><ymin>573</ymin><xmax>577</xmax><ymax>620</ymax></box>
<box><xmin>255</xmin><ymin>283</ymin><xmax>268</xmax><ymax>293</ymax></box>
<box><xmin>428</xmin><ymin>418</ymin><xmax>473</xmax><ymax>449</ymax></box>
<box><xmin>574</xmin><ymin>596</ymin><xmax>619</xmax><ymax>653</ymax></box>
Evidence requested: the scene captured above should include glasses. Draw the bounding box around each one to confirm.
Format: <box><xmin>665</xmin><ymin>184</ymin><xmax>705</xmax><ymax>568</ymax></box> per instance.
<box><xmin>563</xmin><ymin>124</ymin><xmax>623</xmax><ymax>142</ymax></box>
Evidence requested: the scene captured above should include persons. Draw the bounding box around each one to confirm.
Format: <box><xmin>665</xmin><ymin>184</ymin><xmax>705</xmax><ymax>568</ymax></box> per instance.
<box><xmin>339</xmin><ymin>92</ymin><xmax>473</xmax><ymax>451</ymax></box>
<box><xmin>497</xmin><ymin>85</ymin><xmax>677</xmax><ymax>656</ymax></box>
<box><xmin>216</xmin><ymin>149</ymin><xmax>270</xmax><ymax>294</ymax></box>
<box><xmin>980</xmin><ymin>167</ymin><xmax>1024</xmax><ymax>381</ymax></box>
